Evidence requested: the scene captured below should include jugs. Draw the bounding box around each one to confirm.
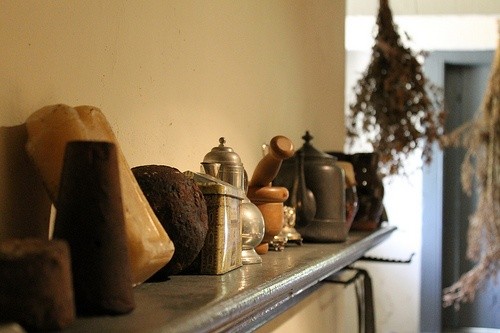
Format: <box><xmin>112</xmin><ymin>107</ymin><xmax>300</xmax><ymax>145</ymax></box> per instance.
<box><xmin>199</xmin><ymin>136</ymin><xmax>265</xmax><ymax>267</ymax></box>
<box><xmin>274</xmin><ymin>128</ymin><xmax>345</xmax><ymax>242</ymax></box>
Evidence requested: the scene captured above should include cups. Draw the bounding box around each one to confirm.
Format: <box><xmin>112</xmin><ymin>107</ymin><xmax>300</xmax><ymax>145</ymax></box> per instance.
<box><xmin>51</xmin><ymin>140</ymin><xmax>135</xmax><ymax>314</ymax></box>
